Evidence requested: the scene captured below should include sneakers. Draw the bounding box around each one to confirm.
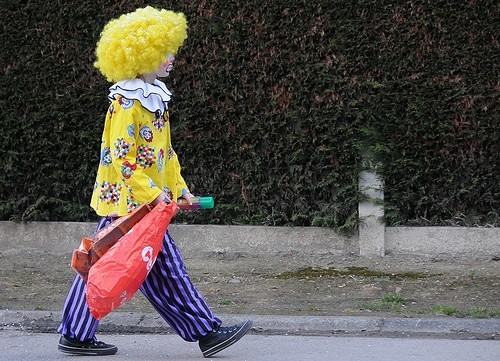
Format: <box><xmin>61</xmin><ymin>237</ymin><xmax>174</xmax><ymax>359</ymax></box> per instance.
<box><xmin>199</xmin><ymin>320</ymin><xmax>253</xmax><ymax>358</ymax></box>
<box><xmin>58</xmin><ymin>335</ymin><xmax>118</xmax><ymax>356</ymax></box>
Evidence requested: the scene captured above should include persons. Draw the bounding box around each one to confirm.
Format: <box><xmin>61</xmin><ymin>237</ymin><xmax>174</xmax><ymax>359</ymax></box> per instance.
<box><xmin>57</xmin><ymin>5</ymin><xmax>255</xmax><ymax>359</ymax></box>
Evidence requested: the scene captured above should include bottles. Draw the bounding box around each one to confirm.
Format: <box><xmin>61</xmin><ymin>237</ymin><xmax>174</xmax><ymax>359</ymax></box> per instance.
<box><xmin>176</xmin><ymin>197</ymin><xmax>215</xmax><ymax>209</ymax></box>
